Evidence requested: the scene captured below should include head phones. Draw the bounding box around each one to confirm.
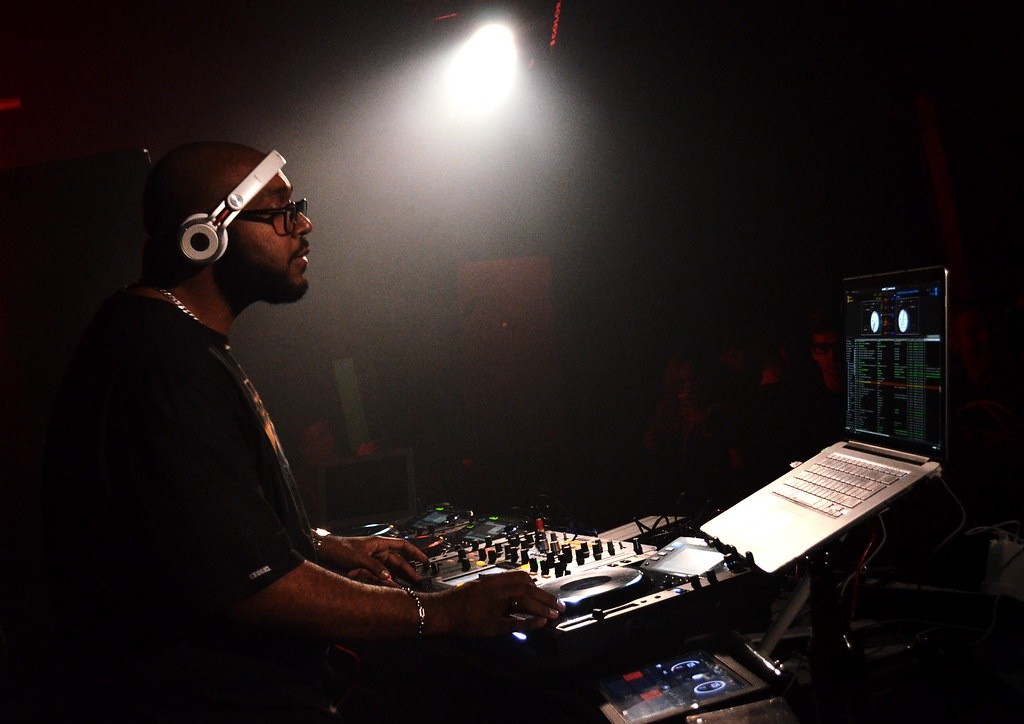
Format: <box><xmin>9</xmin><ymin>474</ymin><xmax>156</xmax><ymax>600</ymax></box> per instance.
<box><xmin>175</xmin><ymin>149</ymin><xmax>287</xmax><ymax>263</ymax></box>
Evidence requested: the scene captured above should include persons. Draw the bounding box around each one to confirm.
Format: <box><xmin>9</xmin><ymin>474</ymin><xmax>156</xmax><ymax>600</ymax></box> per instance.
<box><xmin>288</xmin><ymin>417</ymin><xmax>379</xmax><ymax>523</ymax></box>
<box><xmin>637</xmin><ymin>298</ymin><xmax>1024</xmax><ymax>545</ymax></box>
<box><xmin>49</xmin><ymin>140</ymin><xmax>567</xmax><ymax>723</ymax></box>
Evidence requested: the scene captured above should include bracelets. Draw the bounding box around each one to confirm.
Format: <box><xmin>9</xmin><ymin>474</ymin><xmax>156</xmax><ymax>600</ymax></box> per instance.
<box><xmin>312</xmin><ymin>527</ymin><xmax>332</xmax><ymax>554</ymax></box>
<box><xmin>401</xmin><ymin>586</ymin><xmax>425</xmax><ymax>642</ymax></box>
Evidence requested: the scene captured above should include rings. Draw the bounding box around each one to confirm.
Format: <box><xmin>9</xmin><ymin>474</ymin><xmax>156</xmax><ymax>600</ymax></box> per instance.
<box><xmin>509</xmin><ymin>597</ymin><xmax>519</xmax><ymax>614</ymax></box>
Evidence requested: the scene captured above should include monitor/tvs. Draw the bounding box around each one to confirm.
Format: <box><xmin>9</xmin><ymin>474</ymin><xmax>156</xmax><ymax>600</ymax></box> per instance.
<box><xmin>590</xmin><ymin>642</ymin><xmax>768</xmax><ymax>724</ymax></box>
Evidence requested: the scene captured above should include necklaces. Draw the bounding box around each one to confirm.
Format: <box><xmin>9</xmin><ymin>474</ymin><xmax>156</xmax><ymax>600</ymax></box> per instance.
<box><xmin>155</xmin><ymin>284</ymin><xmax>204</xmax><ymax>328</ymax></box>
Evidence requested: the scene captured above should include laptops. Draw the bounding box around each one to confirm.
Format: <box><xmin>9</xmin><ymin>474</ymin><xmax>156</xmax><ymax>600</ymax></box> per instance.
<box><xmin>317</xmin><ymin>447</ymin><xmax>418</xmax><ymax>535</ymax></box>
<box><xmin>699</xmin><ymin>265</ymin><xmax>948</xmax><ymax>573</ymax></box>
